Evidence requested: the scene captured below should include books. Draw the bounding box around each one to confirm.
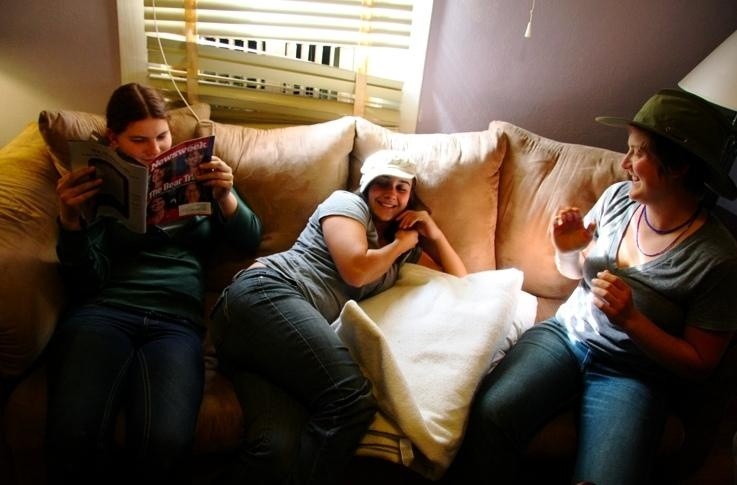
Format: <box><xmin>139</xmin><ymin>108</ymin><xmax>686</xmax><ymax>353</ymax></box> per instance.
<box><xmin>61</xmin><ymin>137</ymin><xmax>212</xmax><ymax>234</ymax></box>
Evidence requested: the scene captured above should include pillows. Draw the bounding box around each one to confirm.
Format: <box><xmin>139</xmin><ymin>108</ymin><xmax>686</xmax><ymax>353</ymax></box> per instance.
<box><xmin>488</xmin><ymin>119</ymin><xmax>633</xmax><ymax>300</ymax></box>
<box><xmin>197</xmin><ymin>115</ymin><xmax>353</xmax><ymax>253</ymax></box>
<box><xmin>353</xmin><ymin>114</ymin><xmax>507</xmax><ymax>274</ymax></box>
<box><xmin>38</xmin><ymin>102</ymin><xmax>210</xmax><ymax>178</ymax></box>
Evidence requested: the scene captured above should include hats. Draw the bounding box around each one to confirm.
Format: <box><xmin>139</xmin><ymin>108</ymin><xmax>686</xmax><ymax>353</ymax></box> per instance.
<box><xmin>595</xmin><ymin>94</ymin><xmax>724</xmax><ymax>162</ymax></box>
<box><xmin>359</xmin><ymin>149</ymin><xmax>417</xmax><ymax>193</ymax></box>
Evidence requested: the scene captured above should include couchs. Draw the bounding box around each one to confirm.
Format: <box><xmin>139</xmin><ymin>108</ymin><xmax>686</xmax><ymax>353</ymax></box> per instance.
<box><xmin>0</xmin><ymin>114</ymin><xmax>641</xmax><ymax>484</ymax></box>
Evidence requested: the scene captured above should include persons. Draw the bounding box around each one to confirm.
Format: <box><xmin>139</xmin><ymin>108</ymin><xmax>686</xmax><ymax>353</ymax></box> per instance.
<box><xmin>452</xmin><ymin>91</ymin><xmax>737</xmax><ymax>482</ymax></box>
<box><xmin>147</xmin><ymin>149</ymin><xmax>206</xmax><ymax>226</ymax></box>
<box><xmin>31</xmin><ymin>82</ymin><xmax>264</xmax><ymax>484</ymax></box>
<box><xmin>211</xmin><ymin>151</ymin><xmax>467</xmax><ymax>485</ymax></box>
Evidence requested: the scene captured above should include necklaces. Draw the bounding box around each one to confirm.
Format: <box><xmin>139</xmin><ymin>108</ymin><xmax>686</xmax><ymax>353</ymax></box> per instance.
<box><xmin>635</xmin><ymin>202</ymin><xmax>702</xmax><ymax>258</ymax></box>
